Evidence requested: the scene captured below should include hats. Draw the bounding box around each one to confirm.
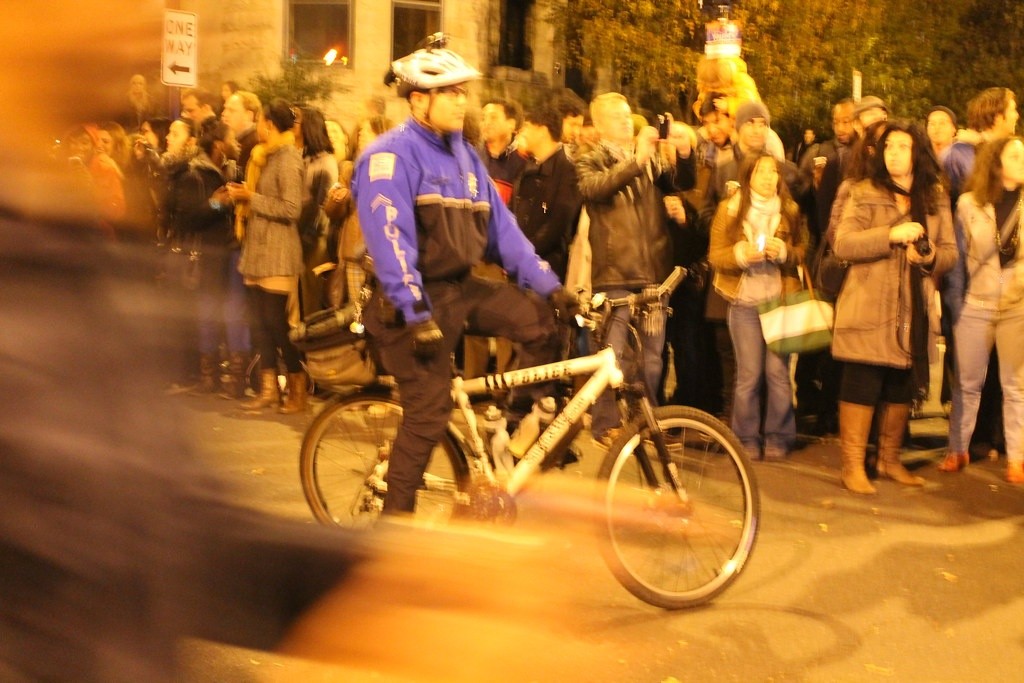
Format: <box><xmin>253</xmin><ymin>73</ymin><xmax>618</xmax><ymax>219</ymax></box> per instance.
<box><xmin>925</xmin><ymin>104</ymin><xmax>957</xmax><ymax>132</ymax></box>
<box><xmin>734</xmin><ymin>100</ymin><xmax>772</xmax><ymax>134</ymax></box>
<box><xmin>855</xmin><ymin>95</ymin><xmax>892</xmax><ymax>117</ymax></box>
<box><xmin>703</xmin><ymin>19</ymin><xmax>743</xmax><ymax>59</ymax></box>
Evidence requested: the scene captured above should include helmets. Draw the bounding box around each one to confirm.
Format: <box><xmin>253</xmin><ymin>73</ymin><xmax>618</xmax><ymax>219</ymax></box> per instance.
<box><xmin>390</xmin><ymin>47</ymin><xmax>481</xmax><ymax>97</ymax></box>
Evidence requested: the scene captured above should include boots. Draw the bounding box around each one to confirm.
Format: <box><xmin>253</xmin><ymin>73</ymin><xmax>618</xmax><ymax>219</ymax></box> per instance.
<box><xmin>190</xmin><ymin>348</ymin><xmax>222</xmax><ymax>397</ymax></box>
<box><xmin>279</xmin><ymin>372</ymin><xmax>309</xmax><ymax>415</ymax></box>
<box><xmin>219</xmin><ymin>351</ymin><xmax>245</xmax><ymax>399</ymax></box>
<box><xmin>241</xmin><ymin>367</ymin><xmax>282</xmax><ymax>409</ymax></box>
<box><xmin>873</xmin><ymin>402</ymin><xmax>926</xmax><ymax>486</ymax></box>
<box><xmin>838</xmin><ymin>398</ymin><xmax>877</xmax><ymax>495</ymax></box>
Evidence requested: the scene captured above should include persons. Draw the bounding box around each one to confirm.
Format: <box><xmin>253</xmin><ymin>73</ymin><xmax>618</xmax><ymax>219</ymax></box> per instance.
<box><xmin>454</xmin><ymin>53</ymin><xmax>1024</xmax><ymax>494</ymax></box>
<box><xmin>350</xmin><ymin>33</ymin><xmax>582</xmax><ymax>529</ymax></box>
<box><xmin>44</xmin><ymin>72</ymin><xmax>400</xmax><ymax>413</ymax></box>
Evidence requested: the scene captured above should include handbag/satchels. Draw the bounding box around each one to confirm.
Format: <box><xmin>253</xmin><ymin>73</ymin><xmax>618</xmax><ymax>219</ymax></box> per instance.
<box><xmin>755</xmin><ymin>247</ymin><xmax>835</xmax><ymax>353</ymax></box>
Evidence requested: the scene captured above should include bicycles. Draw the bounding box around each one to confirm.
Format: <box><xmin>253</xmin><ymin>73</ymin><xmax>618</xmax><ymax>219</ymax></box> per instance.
<box><xmin>300</xmin><ymin>264</ymin><xmax>763</xmax><ymax>613</ymax></box>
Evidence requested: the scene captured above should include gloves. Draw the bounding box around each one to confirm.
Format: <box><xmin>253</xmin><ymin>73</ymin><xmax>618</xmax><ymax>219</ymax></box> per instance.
<box><xmin>409</xmin><ymin>315</ymin><xmax>445</xmax><ymax>361</ymax></box>
<box><xmin>547</xmin><ymin>281</ymin><xmax>584</xmax><ymax>330</ymax></box>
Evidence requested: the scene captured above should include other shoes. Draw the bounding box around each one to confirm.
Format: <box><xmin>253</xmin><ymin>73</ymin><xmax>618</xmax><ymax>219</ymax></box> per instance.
<box><xmin>645</xmin><ymin>430</ymin><xmax>682</xmax><ymax>451</ymax></box>
<box><xmin>762</xmin><ymin>436</ymin><xmax>786</xmax><ymax>461</ymax></box>
<box><xmin>739</xmin><ymin>440</ymin><xmax>763</xmax><ymax>460</ymax></box>
<box><xmin>591</xmin><ymin>425</ymin><xmax>623</xmax><ymax>448</ymax></box>
<box><xmin>510</xmin><ymin>446</ymin><xmax>577</xmax><ymax>472</ymax></box>
<box><xmin>1005</xmin><ymin>458</ymin><xmax>1023</xmax><ymax>482</ymax></box>
<box><xmin>936</xmin><ymin>449</ymin><xmax>971</xmax><ymax>471</ymax></box>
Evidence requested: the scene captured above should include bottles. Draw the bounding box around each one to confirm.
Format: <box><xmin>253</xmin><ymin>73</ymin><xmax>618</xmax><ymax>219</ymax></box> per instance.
<box><xmin>511</xmin><ymin>396</ymin><xmax>556</xmax><ymax>459</ymax></box>
<box><xmin>483</xmin><ymin>407</ymin><xmax>515</xmax><ymax>476</ymax></box>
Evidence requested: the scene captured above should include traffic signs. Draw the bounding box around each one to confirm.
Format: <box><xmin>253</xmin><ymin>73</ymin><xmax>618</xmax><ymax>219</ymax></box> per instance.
<box><xmin>160</xmin><ymin>8</ymin><xmax>198</xmax><ymax>88</ymax></box>
